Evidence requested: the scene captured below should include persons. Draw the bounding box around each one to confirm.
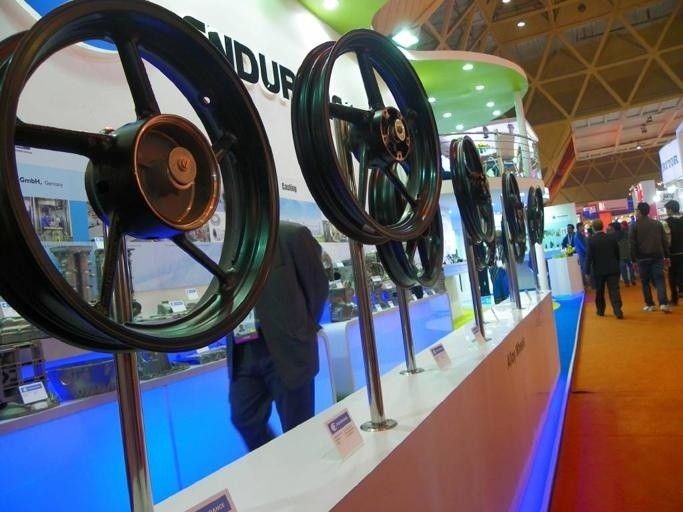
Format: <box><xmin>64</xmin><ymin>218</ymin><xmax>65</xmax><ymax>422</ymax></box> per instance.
<box><xmin>226</xmin><ymin>220</ymin><xmax>330</xmax><ymax>452</ymax></box>
<box><xmin>562</xmin><ymin>200</ymin><xmax>683</xmax><ymax>320</ymax></box>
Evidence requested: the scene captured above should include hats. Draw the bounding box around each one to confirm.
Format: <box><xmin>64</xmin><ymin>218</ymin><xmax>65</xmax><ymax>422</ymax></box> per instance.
<box><xmin>665</xmin><ymin>200</ymin><xmax>678</xmax><ymax>210</ymax></box>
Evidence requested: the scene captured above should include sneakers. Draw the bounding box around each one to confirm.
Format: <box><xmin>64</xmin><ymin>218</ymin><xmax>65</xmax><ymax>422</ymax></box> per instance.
<box><xmin>616</xmin><ymin>311</ymin><xmax>623</xmax><ymax>319</ymax></box>
<box><xmin>669</xmin><ymin>299</ymin><xmax>677</xmax><ymax>304</ymax></box>
<box><xmin>625</xmin><ymin>279</ymin><xmax>636</xmax><ymax>287</ymax></box>
<box><xmin>660</xmin><ymin>304</ymin><xmax>671</xmax><ymax>313</ymax></box>
<box><xmin>678</xmin><ymin>290</ymin><xmax>683</xmax><ymax>297</ymax></box>
<box><xmin>643</xmin><ymin>305</ymin><xmax>656</xmax><ymax>311</ymax></box>
<box><xmin>596</xmin><ymin>310</ymin><xmax>603</xmax><ymax>315</ymax></box>
